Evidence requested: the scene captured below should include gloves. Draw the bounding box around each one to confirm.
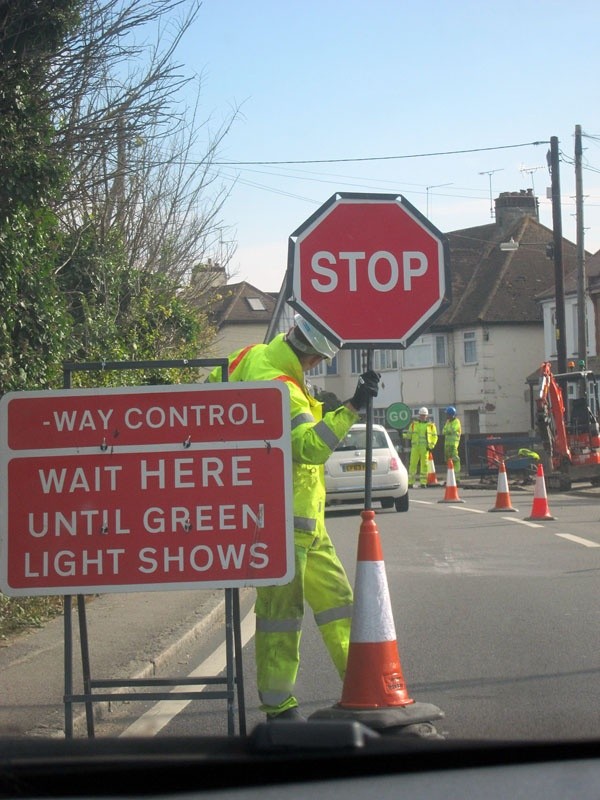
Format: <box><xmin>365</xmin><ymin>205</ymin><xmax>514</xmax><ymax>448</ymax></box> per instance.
<box><xmin>351</xmin><ymin>369</ymin><xmax>382</xmax><ymax>412</ymax></box>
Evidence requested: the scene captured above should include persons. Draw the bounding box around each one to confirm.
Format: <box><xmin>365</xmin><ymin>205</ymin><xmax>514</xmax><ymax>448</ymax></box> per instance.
<box><xmin>204</xmin><ymin>313</ymin><xmax>382</xmax><ymax>722</ymax></box>
<box><xmin>442</xmin><ymin>406</ymin><xmax>461</xmax><ymax>485</ymax></box>
<box><xmin>399</xmin><ymin>407</ymin><xmax>438</xmax><ymax>488</ymax></box>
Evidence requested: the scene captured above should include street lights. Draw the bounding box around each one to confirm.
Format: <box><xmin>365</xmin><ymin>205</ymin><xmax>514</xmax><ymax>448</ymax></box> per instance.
<box><xmin>479</xmin><ymin>169</ymin><xmax>503</xmax><ymax>218</ymax></box>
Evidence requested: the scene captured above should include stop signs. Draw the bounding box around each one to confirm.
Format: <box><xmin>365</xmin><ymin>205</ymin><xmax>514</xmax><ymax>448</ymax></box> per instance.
<box><xmin>284</xmin><ymin>192</ymin><xmax>455</xmax><ymax>348</ymax></box>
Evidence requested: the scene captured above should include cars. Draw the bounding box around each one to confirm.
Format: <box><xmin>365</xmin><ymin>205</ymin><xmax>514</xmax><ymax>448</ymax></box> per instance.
<box><xmin>322</xmin><ymin>421</ymin><xmax>411</xmax><ymax>512</ymax></box>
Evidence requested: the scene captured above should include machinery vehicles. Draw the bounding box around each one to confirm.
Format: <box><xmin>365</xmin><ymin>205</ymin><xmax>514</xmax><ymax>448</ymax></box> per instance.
<box><xmin>523</xmin><ymin>360</ymin><xmax>600</xmax><ymax>490</ymax></box>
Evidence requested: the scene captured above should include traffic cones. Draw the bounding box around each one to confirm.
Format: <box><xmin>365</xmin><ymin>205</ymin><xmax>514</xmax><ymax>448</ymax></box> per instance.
<box><xmin>308</xmin><ymin>511</ymin><xmax>448</xmax><ymax>733</ymax></box>
<box><xmin>424</xmin><ymin>452</ymin><xmax>443</xmax><ymax>488</ymax></box>
<box><xmin>486</xmin><ymin>458</ymin><xmax>517</xmax><ymax>513</ymax></box>
<box><xmin>521</xmin><ymin>463</ymin><xmax>560</xmax><ymax>521</ymax></box>
<box><xmin>439</xmin><ymin>458</ymin><xmax>465</xmax><ymax>503</ymax></box>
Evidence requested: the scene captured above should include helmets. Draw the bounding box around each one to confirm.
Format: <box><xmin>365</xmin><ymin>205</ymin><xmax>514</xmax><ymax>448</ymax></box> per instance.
<box><xmin>419</xmin><ymin>407</ymin><xmax>428</xmax><ymax>415</ymax></box>
<box><xmin>445</xmin><ymin>407</ymin><xmax>456</xmax><ymax>415</ymax></box>
<box><xmin>294</xmin><ymin>313</ymin><xmax>340</xmax><ymax>359</ymax></box>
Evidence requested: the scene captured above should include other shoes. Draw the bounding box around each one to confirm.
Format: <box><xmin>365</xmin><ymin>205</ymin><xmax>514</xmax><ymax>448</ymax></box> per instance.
<box><xmin>266</xmin><ymin>706</ymin><xmax>309</xmax><ymax>725</ymax></box>
